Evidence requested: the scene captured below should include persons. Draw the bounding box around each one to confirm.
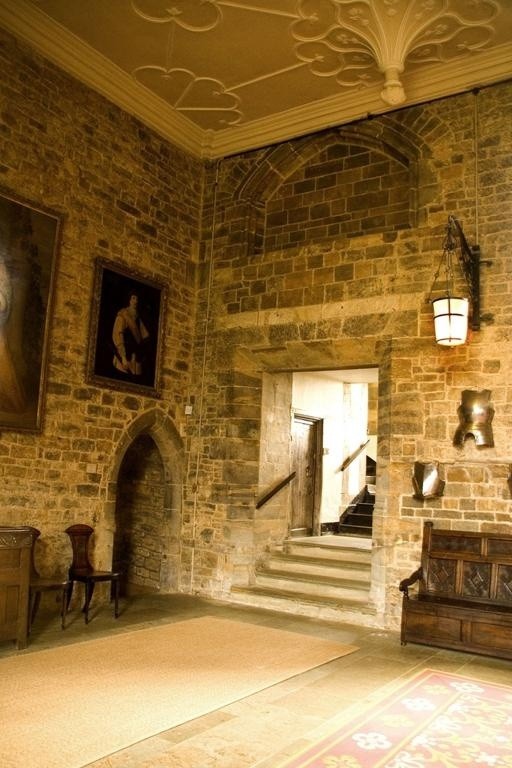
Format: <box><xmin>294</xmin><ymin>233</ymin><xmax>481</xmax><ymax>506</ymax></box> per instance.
<box><xmin>110</xmin><ymin>290</ymin><xmax>155</xmax><ymax>383</ymax></box>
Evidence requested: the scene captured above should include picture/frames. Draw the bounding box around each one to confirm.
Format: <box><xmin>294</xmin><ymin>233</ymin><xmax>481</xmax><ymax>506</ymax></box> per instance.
<box><xmin>0</xmin><ymin>185</ymin><xmax>66</xmax><ymax>434</ymax></box>
<box><xmin>85</xmin><ymin>256</ymin><xmax>171</xmax><ymax>400</ymax></box>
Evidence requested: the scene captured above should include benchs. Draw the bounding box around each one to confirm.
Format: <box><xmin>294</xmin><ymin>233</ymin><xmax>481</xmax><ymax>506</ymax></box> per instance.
<box><xmin>399</xmin><ymin>521</ymin><xmax>512</xmax><ymax>661</ymax></box>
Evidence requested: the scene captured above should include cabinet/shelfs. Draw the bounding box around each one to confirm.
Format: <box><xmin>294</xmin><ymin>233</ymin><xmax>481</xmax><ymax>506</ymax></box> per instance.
<box><xmin>0</xmin><ymin>526</ymin><xmax>34</xmax><ymax>650</ymax></box>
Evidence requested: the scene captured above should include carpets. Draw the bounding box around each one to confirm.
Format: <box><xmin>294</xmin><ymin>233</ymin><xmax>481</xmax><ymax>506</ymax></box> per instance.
<box><xmin>0</xmin><ymin>615</ymin><xmax>360</xmax><ymax>768</ymax></box>
<box><xmin>277</xmin><ymin>667</ymin><xmax>512</xmax><ymax>768</ymax></box>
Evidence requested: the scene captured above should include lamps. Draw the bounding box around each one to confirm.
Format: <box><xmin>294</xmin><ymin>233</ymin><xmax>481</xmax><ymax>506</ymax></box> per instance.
<box><xmin>426</xmin><ymin>214</ymin><xmax>481</xmax><ymax>348</ymax></box>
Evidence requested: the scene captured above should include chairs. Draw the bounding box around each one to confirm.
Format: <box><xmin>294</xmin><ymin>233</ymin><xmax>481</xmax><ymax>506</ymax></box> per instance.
<box><xmin>64</xmin><ymin>524</ymin><xmax>121</xmax><ymax>624</ymax></box>
<box><xmin>18</xmin><ymin>526</ymin><xmax>70</xmax><ymax>634</ymax></box>
